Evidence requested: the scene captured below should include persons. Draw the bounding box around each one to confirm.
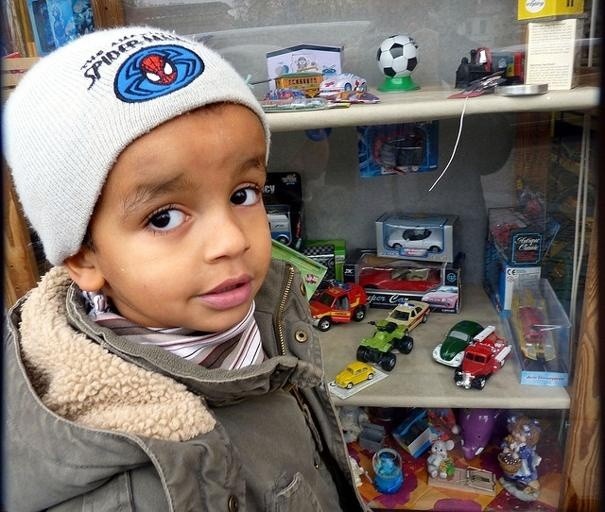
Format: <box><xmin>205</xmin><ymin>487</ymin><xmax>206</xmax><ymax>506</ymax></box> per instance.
<box><xmin>1</xmin><ymin>24</ymin><xmax>373</xmax><ymax>512</ymax></box>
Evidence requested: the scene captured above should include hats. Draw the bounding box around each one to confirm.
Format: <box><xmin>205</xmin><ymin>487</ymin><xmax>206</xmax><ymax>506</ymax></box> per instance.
<box><xmin>2</xmin><ymin>25</ymin><xmax>272</xmax><ymax>269</ymax></box>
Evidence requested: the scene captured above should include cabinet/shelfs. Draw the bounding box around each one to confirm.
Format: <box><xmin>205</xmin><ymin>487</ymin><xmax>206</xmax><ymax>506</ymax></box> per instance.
<box><xmin>250</xmin><ymin>73</ymin><xmax>605</xmax><ymax>512</ymax></box>
